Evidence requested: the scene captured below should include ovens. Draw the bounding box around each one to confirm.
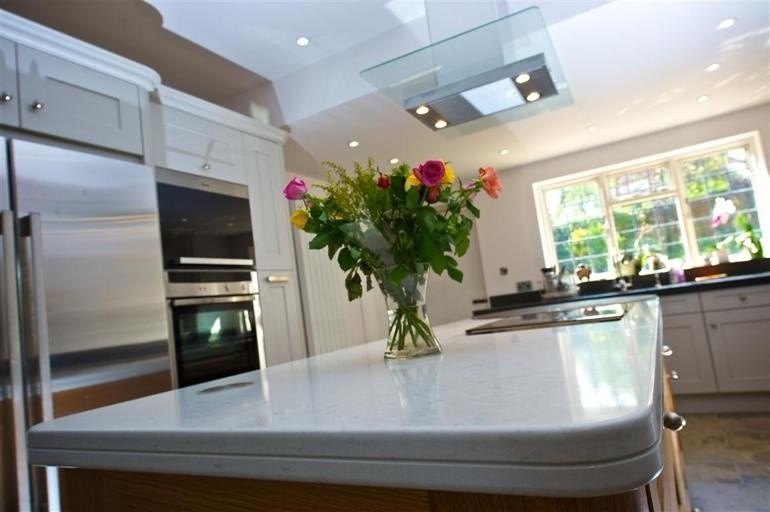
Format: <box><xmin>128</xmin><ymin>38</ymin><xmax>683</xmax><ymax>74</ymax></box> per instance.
<box><xmin>154</xmin><ymin>166</ymin><xmax>271</xmax><ymax>390</ymax></box>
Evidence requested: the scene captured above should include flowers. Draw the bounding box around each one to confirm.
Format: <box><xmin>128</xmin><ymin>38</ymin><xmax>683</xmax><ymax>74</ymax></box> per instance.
<box><xmin>283</xmin><ymin>156</ymin><xmax>505</xmax><ymax>350</ymax></box>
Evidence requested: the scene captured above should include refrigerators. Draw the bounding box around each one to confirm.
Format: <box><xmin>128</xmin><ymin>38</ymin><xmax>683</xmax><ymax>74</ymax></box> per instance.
<box><xmin>1</xmin><ymin>130</ymin><xmax>179</xmax><ymax>512</ymax></box>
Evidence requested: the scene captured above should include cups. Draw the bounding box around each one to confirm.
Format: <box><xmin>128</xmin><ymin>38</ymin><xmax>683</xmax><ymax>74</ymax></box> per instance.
<box><xmin>668</xmin><ymin>264</ymin><xmax>685</xmax><ymax>283</ymax></box>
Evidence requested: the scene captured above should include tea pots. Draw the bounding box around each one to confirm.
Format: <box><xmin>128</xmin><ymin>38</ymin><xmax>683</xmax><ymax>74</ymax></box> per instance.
<box><xmin>540</xmin><ymin>266</ymin><xmax>560</xmax><ymax>294</ymax></box>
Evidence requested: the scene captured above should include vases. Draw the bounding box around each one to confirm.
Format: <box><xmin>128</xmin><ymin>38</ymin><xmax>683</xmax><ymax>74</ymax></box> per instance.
<box><xmin>372</xmin><ymin>261</ymin><xmax>444</xmax><ymax>358</ymax></box>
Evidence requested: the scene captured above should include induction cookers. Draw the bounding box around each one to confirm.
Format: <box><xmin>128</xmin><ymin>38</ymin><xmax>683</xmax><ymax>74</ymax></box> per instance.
<box><xmin>466</xmin><ymin>302</ymin><xmax>636</xmax><ymax>337</ymax></box>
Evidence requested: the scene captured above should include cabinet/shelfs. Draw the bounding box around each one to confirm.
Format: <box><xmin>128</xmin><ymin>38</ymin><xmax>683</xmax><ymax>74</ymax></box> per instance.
<box><xmin>660</xmin><ymin>284</ymin><xmax>769</xmax><ymax>417</ymax></box>
<box><xmin>1</xmin><ymin>10</ymin><xmax>162</xmax><ymax>161</ymax></box>
<box><xmin>25</xmin><ymin>292</ymin><xmax>689</xmax><ymax>512</ymax></box>
